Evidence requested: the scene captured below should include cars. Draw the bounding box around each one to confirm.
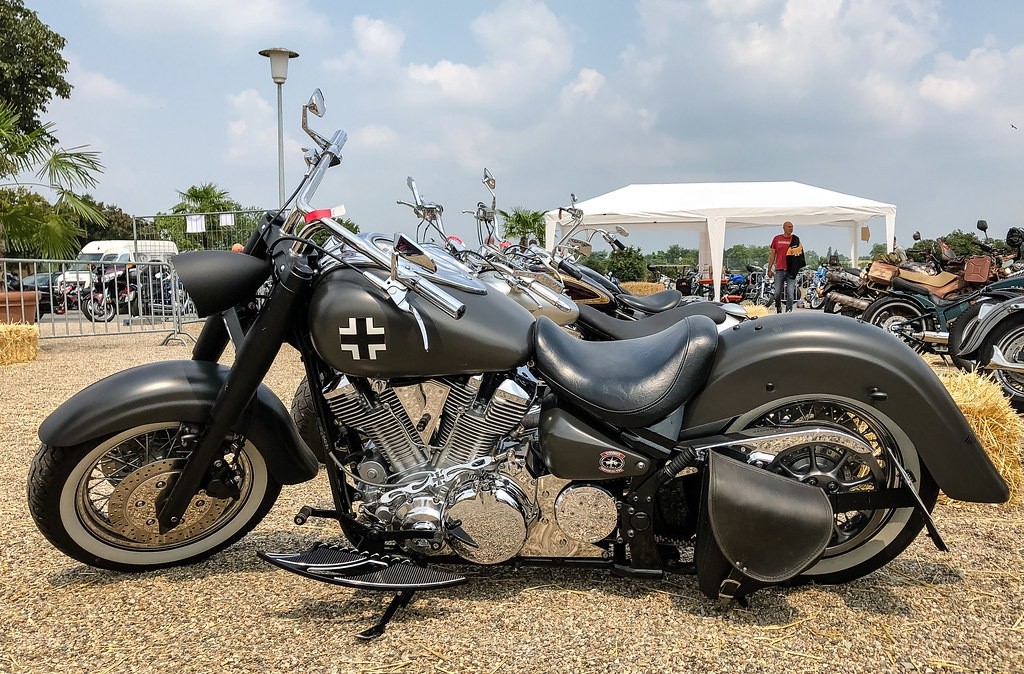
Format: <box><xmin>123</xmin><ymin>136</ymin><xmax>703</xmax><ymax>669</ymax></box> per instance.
<box><xmin>20</xmin><ymin>272</ymin><xmax>65</xmax><ymax>314</ymax></box>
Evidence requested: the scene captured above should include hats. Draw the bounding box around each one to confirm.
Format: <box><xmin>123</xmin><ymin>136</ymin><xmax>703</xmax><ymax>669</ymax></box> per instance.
<box><xmin>232</xmin><ymin>242</ymin><xmax>245</xmax><ymax>252</ymax></box>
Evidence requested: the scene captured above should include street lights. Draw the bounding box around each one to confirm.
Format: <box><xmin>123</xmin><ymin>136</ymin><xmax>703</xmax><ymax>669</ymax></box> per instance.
<box><xmin>258</xmin><ymin>46</ymin><xmax>300</xmax><ymax>211</ymax></box>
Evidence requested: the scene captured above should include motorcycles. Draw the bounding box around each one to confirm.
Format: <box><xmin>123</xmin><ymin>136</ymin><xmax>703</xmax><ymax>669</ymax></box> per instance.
<box><xmin>76</xmin><ymin>258</ymin><xmax>176</xmax><ymax>324</ymax></box>
<box><xmin>54</xmin><ymin>281</ymin><xmax>94</xmax><ymax>314</ymax></box>
<box><xmin>24</xmin><ymin>88</ymin><xmax>1024</xmax><ymax>641</ymax></box>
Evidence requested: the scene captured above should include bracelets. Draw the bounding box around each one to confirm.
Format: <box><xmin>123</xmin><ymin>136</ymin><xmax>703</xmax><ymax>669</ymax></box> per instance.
<box><xmin>768</xmin><ymin>270</ymin><xmax>771</xmax><ymax>272</ymax></box>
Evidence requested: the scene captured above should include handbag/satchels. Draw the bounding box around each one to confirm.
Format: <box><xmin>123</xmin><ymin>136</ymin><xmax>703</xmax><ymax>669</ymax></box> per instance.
<box><xmin>695</xmin><ymin>448</ymin><xmax>834</xmax><ymax>606</ymax></box>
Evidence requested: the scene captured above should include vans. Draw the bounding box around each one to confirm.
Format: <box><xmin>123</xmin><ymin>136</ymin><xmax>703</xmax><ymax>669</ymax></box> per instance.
<box><xmin>54</xmin><ymin>240</ymin><xmax>178</xmax><ymax>292</ymax></box>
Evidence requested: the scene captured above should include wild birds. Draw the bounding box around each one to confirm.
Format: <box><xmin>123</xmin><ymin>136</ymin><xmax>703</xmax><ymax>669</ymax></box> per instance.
<box><xmin>1010</xmin><ymin>123</ymin><xmax>1017</xmax><ymax>129</ymax></box>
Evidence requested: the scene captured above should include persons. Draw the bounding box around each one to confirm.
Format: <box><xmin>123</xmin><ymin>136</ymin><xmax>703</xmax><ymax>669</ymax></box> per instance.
<box><xmin>893</xmin><ymin>236</ymin><xmax>907</xmax><ymax>262</ymax></box>
<box><xmin>483</xmin><ymin>237</ymin><xmax>537</xmax><ymax>253</ymax></box>
<box><xmin>767</xmin><ymin>221</ymin><xmax>800</xmax><ymax>313</ymax></box>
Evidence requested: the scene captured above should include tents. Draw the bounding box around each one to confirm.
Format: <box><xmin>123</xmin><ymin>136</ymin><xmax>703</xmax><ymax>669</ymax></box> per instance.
<box><xmin>545</xmin><ymin>181</ymin><xmax>897</xmax><ymax>305</ymax></box>
